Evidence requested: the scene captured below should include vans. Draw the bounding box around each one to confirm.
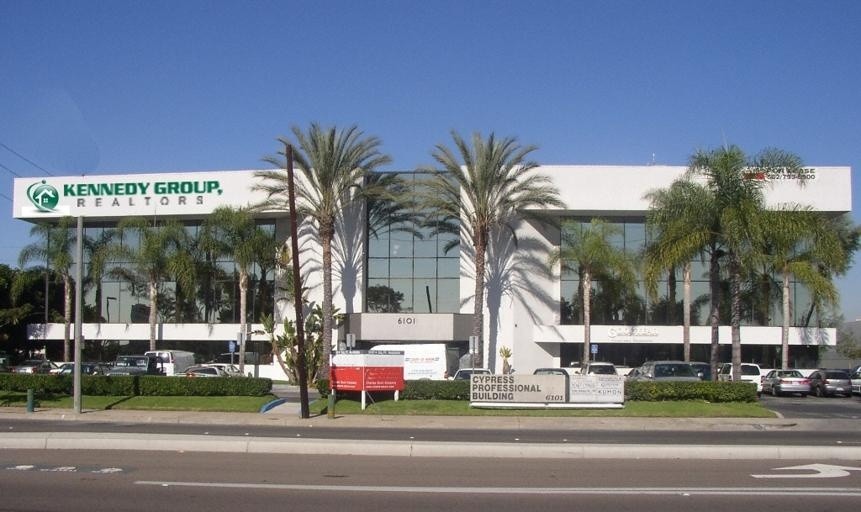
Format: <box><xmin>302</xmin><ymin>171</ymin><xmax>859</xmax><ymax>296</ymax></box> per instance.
<box><xmin>576</xmin><ymin>362</ymin><xmax>618</xmax><ymax>375</ymax></box>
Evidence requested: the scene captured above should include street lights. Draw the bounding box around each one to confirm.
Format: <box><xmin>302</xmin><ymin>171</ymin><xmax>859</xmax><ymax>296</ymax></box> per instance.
<box><xmin>105</xmin><ymin>296</ymin><xmax>118</xmax><ymax>322</ymax></box>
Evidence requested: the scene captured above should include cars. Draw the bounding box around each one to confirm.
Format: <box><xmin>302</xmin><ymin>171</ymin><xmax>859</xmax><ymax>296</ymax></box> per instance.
<box><xmin>447</xmin><ymin>367</ymin><xmax>494</xmax><ymax>381</ymax></box>
<box><xmin>624</xmin><ymin>361</ymin><xmax>861</xmax><ymax>398</ymax></box>
<box><xmin>1</xmin><ymin>349</ymin><xmax>247</xmax><ymax>380</ymax></box>
<box><xmin>534</xmin><ymin>367</ymin><xmax>569</xmax><ymax>376</ymax></box>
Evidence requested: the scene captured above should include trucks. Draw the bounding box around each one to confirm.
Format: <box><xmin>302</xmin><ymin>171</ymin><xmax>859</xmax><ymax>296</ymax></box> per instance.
<box><xmin>367</xmin><ymin>343</ymin><xmax>461</xmax><ymax>382</ymax></box>
<box><xmin>215</xmin><ymin>352</ymin><xmax>260</xmax><ymax>364</ymax></box>
